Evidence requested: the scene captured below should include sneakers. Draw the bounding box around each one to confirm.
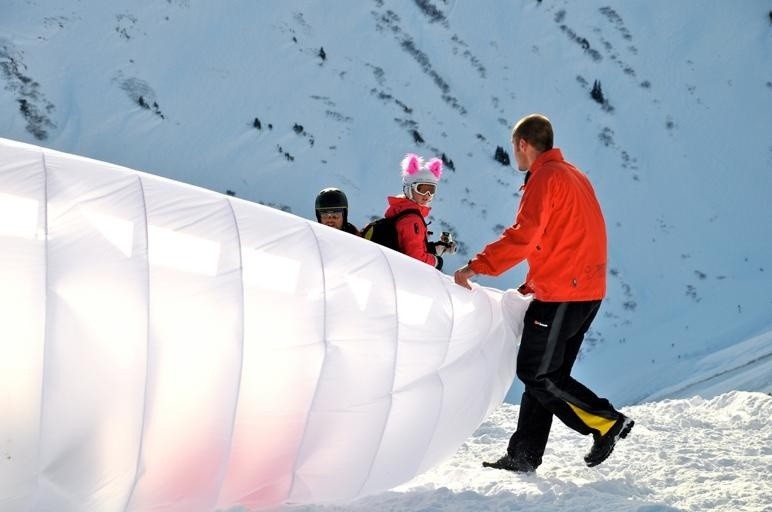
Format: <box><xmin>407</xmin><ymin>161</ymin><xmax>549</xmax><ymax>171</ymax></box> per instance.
<box><xmin>482</xmin><ymin>454</ymin><xmax>535</xmax><ymax>472</ymax></box>
<box><xmin>584</xmin><ymin>413</ymin><xmax>634</xmax><ymax>467</ymax></box>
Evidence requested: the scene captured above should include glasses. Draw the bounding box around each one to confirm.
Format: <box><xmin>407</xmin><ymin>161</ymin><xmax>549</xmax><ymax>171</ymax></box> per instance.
<box><xmin>403</xmin><ymin>178</ymin><xmax>438</xmax><ymax>196</ymax></box>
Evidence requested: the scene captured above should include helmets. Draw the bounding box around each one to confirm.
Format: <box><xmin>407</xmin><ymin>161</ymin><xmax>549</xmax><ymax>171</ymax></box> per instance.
<box><xmin>315</xmin><ymin>187</ymin><xmax>348</xmax><ymax>223</ymax></box>
<box><xmin>401</xmin><ymin>166</ymin><xmax>439</xmax><ymax>200</ymax></box>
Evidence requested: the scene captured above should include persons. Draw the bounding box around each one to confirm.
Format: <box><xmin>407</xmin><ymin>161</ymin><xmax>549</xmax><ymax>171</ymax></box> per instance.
<box><xmin>454</xmin><ymin>113</ymin><xmax>635</xmax><ymax>473</ymax></box>
<box><xmin>314</xmin><ymin>187</ymin><xmax>361</xmax><ymax>236</ymax></box>
<box><xmin>359</xmin><ymin>154</ymin><xmax>458</xmax><ymax>271</ymax></box>
